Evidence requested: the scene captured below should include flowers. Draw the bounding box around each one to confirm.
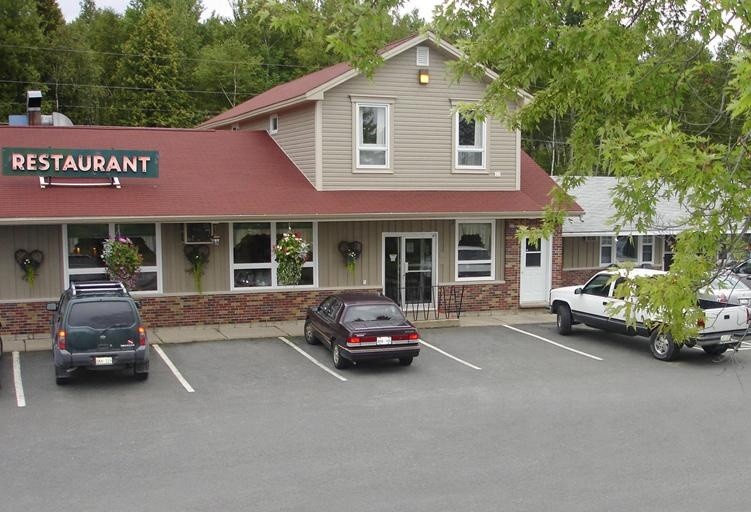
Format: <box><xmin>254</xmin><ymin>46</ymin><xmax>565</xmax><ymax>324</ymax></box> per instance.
<box><xmin>103</xmin><ymin>237</ymin><xmax>143</xmax><ymax>291</ymax></box>
<box><xmin>273</xmin><ymin>223</ymin><xmax>313</xmax><ymax>286</ymax></box>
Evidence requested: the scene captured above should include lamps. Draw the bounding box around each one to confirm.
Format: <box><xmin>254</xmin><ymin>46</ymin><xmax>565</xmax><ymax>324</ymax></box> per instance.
<box><xmin>419</xmin><ymin>69</ymin><xmax>429</xmax><ymax>84</ymax></box>
<box><xmin>210</xmin><ymin>224</ymin><xmax>224</xmax><ymax>247</ymax></box>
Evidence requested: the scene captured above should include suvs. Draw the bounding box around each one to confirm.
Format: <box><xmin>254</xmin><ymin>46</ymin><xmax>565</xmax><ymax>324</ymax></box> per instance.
<box><xmin>73</xmin><ymin>238</ymin><xmax>156</xmax><ymax>289</ymax></box>
<box><xmin>46</xmin><ymin>280</ymin><xmax>149</xmax><ymax>385</ymax></box>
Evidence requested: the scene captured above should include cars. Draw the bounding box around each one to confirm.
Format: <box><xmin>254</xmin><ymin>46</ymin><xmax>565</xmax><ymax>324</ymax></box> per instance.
<box><xmin>305</xmin><ymin>292</ymin><xmax>421</xmax><ymax>369</ymax></box>
<box><xmin>234</xmin><ymin>233</ymin><xmax>313</xmax><ymax>287</ymax></box>
<box><xmin>697</xmin><ymin>257</ymin><xmax>751</xmax><ymax>335</ymax></box>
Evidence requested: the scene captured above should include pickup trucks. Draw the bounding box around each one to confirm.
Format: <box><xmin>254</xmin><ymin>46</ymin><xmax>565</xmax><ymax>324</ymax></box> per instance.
<box><xmin>549</xmin><ymin>268</ymin><xmax>750</xmax><ymax>361</ymax></box>
